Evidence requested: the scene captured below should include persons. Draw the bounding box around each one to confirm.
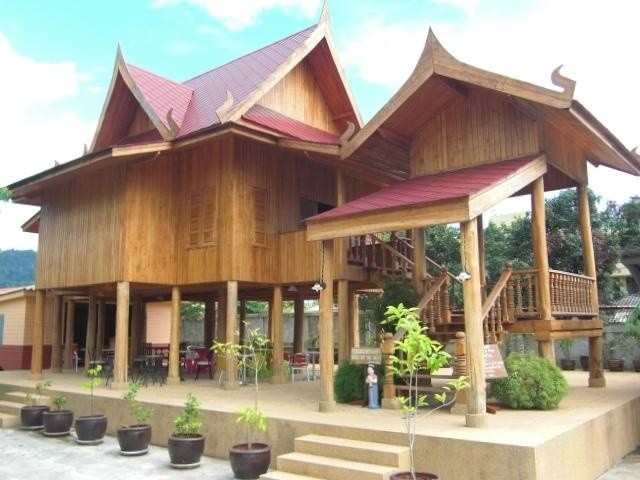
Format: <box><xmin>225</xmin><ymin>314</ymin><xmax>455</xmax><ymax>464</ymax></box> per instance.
<box><xmin>365</xmin><ymin>363</ymin><xmax>380</xmax><ymax>409</ymax></box>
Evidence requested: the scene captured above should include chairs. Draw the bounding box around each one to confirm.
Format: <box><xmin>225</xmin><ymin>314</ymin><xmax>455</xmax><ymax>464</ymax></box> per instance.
<box><xmin>194</xmin><ymin>352</ymin><xmax>213</xmax><ymax>381</ymax></box>
<box><xmin>291</xmin><ymin>352</ymin><xmax>310</xmax><ymax>383</ymax></box>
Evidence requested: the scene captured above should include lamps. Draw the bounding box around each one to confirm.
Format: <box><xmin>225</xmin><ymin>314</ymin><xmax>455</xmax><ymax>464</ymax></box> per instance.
<box><xmin>456</xmin><ymin>223</ymin><xmax>472</xmax><ymax>283</ymax></box>
<box><xmin>311</xmin><ymin>241</ymin><xmax>326</xmax><ymax>292</ymax></box>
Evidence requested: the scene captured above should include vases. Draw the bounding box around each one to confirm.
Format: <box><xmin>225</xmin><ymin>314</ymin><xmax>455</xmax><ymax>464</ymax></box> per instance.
<box><xmin>581</xmin><ymin>355</ymin><xmax>590</xmax><ymax>371</ymax></box>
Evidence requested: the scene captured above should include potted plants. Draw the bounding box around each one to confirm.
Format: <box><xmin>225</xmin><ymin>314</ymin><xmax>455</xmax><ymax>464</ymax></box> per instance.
<box><xmin>116</xmin><ymin>380</ymin><xmax>154</xmax><ymax>456</ymax></box>
<box><xmin>606</xmin><ymin>333</ymin><xmax>637</xmax><ymax>372</ymax></box>
<box><xmin>20</xmin><ymin>381</ymin><xmax>52</xmax><ymax>431</ymax></box>
<box><xmin>623</xmin><ymin>324</ymin><xmax>640</xmax><ymax>372</ymax></box>
<box><xmin>168</xmin><ymin>393</ymin><xmax>205</xmax><ymax>468</ymax></box>
<box><xmin>210</xmin><ymin>320</ymin><xmax>271</xmax><ymax>479</ymax></box>
<box><xmin>379</xmin><ymin>303</ymin><xmax>471</xmax><ymax>480</ymax></box>
<box><xmin>42</xmin><ymin>395</ymin><xmax>74</xmax><ymax>437</ymax></box>
<box><xmin>560</xmin><ymin>339</ymin><xmax>575</xmax><ymax>370</ymax></box>
<box><xmin>75</xmin><ymin>365</ymin><xmax>108</xmax><ymax>444</ymax></box>
